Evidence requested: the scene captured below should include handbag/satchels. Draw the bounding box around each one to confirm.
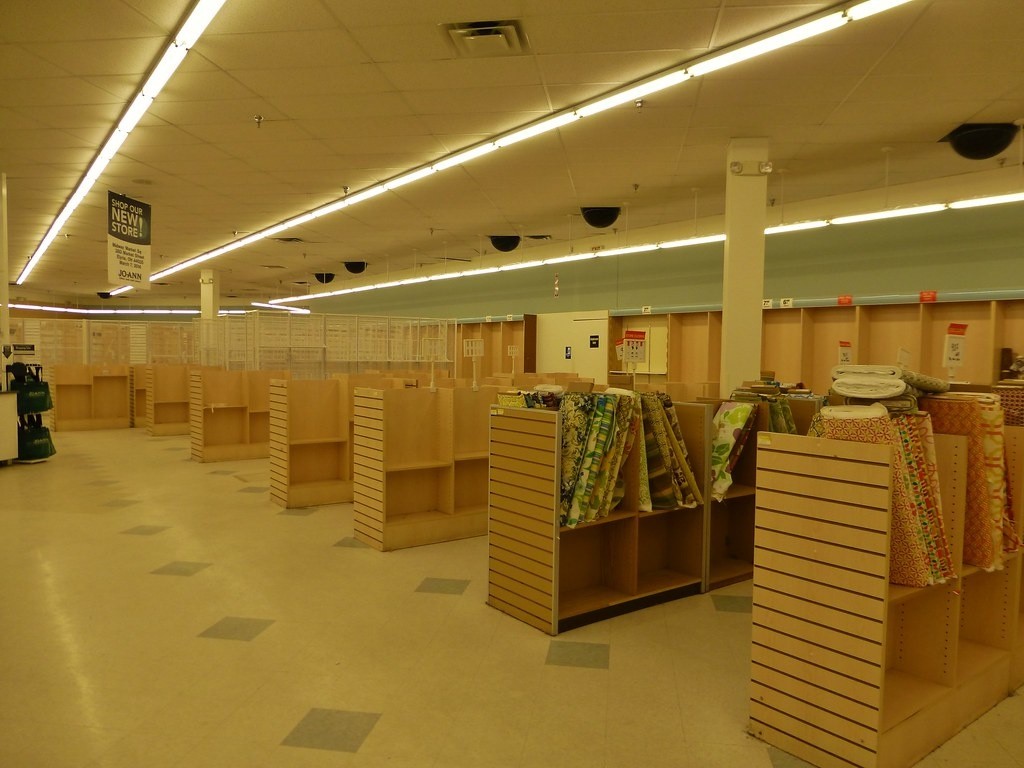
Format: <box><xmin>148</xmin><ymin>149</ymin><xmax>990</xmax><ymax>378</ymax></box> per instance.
<box><xmin>18</xmin><ymin>367</ymin><xmax>53</xmax><ymax>416</ymax></box>
<box><xmin>18</xmin><ymin>414</ymin><xmax>57</xmax><ymax>461</ymax></box>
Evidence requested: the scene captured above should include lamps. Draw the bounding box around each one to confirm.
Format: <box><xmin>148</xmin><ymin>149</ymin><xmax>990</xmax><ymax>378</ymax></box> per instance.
<box><xmin>6</xmin><ymin>0</ymin><xmax>1024</xmax><ymax>313</ymax></box>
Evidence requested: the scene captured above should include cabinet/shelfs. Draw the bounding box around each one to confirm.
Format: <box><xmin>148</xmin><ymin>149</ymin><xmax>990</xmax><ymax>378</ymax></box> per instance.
<box><xmin>749</xmin><ymin>426</ymin><xmax>1024</xmax><ymax>768</ymax></box>
<box><xmin>130</xmin><ymin>364</ymin><xmax>651</xmax><ymax>552</ymax></box>
<box><xmin>486</xmin><ymin>395</ymin><xmax>827</xmax><ymax>638</ymax></box>
